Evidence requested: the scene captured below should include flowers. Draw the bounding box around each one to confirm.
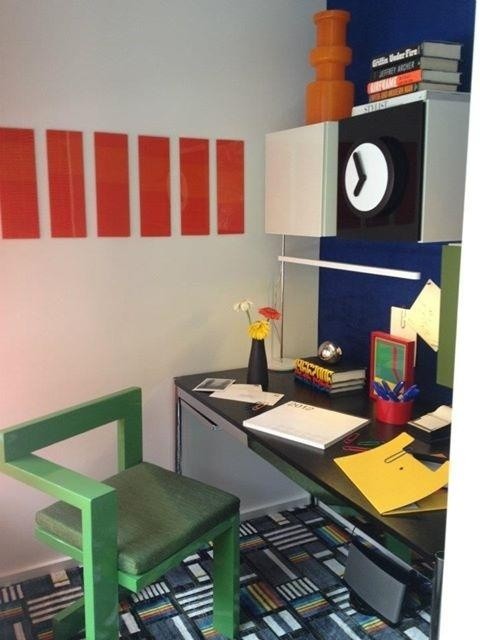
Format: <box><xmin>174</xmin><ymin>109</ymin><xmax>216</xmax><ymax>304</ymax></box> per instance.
<box><xmin>234</xmin><ymin>299</ymin><xmax>281</xmax><ymax>340</ymax></box>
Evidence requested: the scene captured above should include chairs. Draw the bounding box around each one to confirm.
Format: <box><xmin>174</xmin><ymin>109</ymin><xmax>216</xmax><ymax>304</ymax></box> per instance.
<box><xmin>0</xmin><ymin>387</ymin><xmax>241</xmax><ymax>640</ymax></box>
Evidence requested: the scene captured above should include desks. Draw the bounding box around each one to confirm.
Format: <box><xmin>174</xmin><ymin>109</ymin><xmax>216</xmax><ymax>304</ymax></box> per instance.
<box><xmin>174</xmin><ymin>367</ymin><xmax>453</xmax><ymax>640</ymax></box>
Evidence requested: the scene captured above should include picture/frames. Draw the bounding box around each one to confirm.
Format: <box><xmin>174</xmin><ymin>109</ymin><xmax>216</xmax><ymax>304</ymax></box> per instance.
<box><xmin>369</xmin><ymin>332</ymin><xmax>415</xmax><ymax>401</ymax></box>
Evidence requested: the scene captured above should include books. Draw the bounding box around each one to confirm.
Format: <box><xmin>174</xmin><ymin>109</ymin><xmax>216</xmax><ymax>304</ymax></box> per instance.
<box><xmin>294</xmin><ymin>356</ymin><xmax>367</xmax><ymax>395</ymax></box>
<box><xmin>351</xmin><ymin>39</ymin><xmax>464</xmax><ymax>103</ymax></box>
<box><xmin>242</xmin><ymin>401</ymin><xmax>370</xmax><ymax>450</ymax></box>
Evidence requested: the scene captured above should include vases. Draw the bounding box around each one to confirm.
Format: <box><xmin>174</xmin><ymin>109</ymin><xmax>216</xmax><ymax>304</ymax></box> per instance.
<box><xmin>247</xmin><ymin>339</ymin><xmax>269</xmax><ymax>388</ymax></box>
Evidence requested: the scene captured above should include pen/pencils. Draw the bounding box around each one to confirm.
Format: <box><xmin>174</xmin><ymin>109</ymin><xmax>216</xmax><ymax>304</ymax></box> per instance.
<box><xmin>372</xmin><ymin>380</ymin><xmax>420</xmax><ymax>403</ymax></box>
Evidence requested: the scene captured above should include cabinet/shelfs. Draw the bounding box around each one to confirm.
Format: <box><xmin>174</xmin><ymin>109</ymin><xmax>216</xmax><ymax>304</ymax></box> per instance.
<box><xmin>337</xmin><ymin>99</ymin><xmax>469</xmax><ymax>244</ymax></box>
<box><xmin>265</xmin><ymin>120</ymin><xmax>338</xmax><ymax>238</ymax></box>
